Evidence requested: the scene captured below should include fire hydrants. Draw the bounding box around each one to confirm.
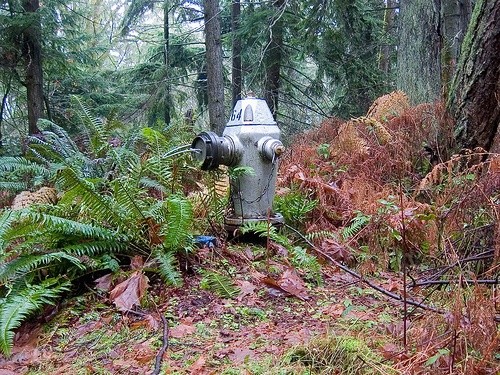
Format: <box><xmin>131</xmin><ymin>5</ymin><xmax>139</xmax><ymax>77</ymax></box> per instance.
<box><xmin>192</xmin><ymin>89</ymin><xmax>286</xmax><ymax>247</ymax></box>
